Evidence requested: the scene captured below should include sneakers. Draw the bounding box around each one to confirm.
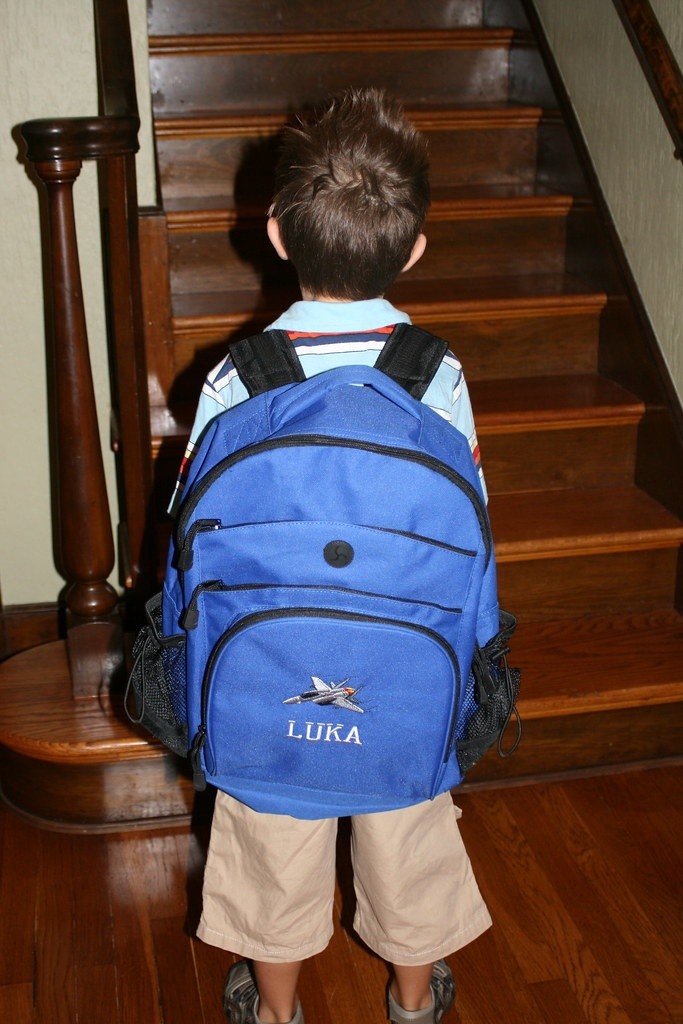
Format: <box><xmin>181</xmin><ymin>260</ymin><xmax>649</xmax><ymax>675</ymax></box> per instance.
<box><xmin>389</xmin><ymin>959</ymin><xmax>456</xmax><ymax>1024</ymax></box>
<box><xmin>224</xmin><ymin>960</ymin><xmax>305</xmax><ymax>1024</ymax></box>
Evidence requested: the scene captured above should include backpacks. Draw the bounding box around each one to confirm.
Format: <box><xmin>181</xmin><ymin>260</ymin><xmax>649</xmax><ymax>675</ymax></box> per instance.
<box><xmin>123</xmin><ymin>323</ymin><xmax>523</xmax><ymax>821</ymax></box>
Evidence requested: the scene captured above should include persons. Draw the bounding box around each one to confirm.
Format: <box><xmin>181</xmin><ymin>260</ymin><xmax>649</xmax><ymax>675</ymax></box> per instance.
<box><xmin>167</xmin><ymin>87</ymin><xmax>494</xmax><ymax>1023</ymax></box>
